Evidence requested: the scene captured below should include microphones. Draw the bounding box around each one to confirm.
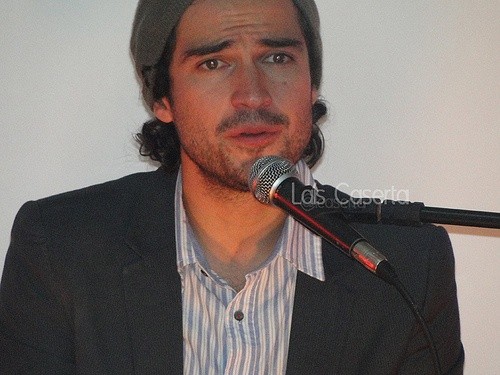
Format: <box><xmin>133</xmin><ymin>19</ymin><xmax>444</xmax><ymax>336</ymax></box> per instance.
<box><xmin>250</xmin><ymin>157</ymin><xmax>396</xmax><ymax>283</ymax></box>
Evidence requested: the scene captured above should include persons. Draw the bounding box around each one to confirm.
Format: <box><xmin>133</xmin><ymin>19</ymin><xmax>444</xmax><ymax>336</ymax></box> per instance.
<box><xmin>0</xmin><ymin>0</ymin><xmax>464</xmax><ymax>375</ymax></box>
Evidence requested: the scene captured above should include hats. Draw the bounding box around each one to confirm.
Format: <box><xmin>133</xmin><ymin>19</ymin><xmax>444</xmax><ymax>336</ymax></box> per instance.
<box><xmin>130</xmin><ymin>0</ymin><xmax>322</xmax><ymax>112</ymax></box>
